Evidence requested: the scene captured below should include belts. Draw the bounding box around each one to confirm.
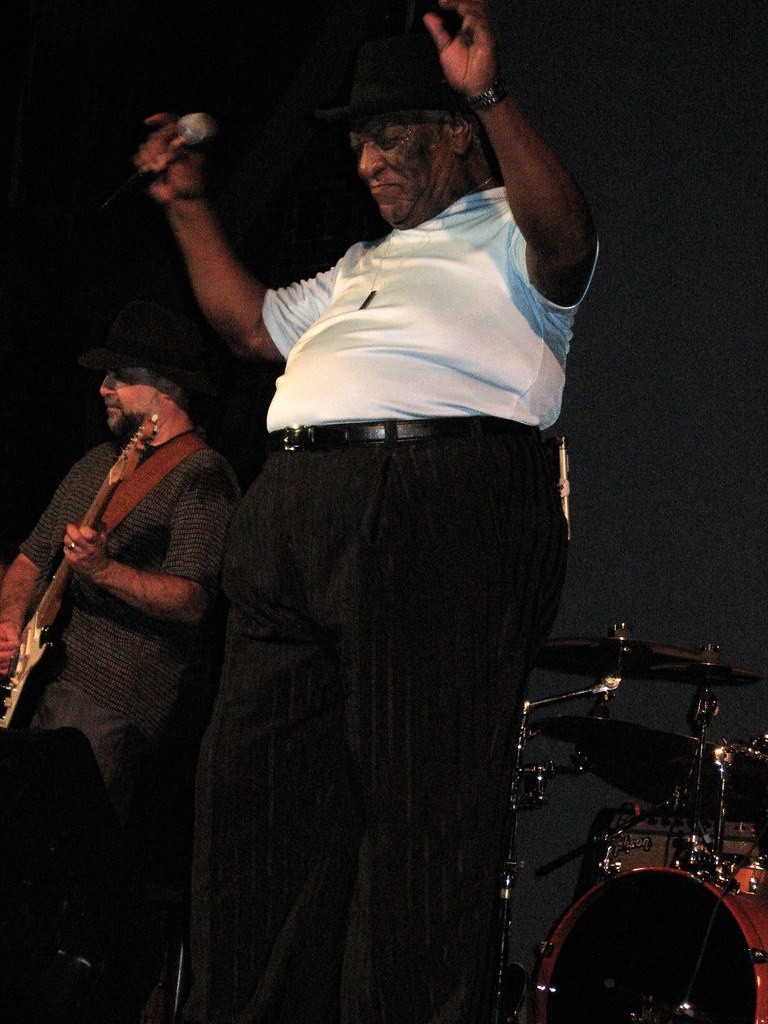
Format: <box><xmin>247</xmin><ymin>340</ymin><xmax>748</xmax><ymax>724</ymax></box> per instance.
<box><xmin>263</xmin><ymin>415</ymin><xmax>522</xmax><ymax>454</ymax></box>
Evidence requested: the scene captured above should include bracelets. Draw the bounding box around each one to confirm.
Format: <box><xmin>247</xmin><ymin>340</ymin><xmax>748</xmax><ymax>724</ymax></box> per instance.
<box><xmin>465</xmin><ymin>83</ymin><xmax>509</xmax><ymax>112</ymax></box>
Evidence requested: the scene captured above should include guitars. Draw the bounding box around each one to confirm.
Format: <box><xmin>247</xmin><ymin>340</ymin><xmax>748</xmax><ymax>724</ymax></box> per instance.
<box><xmin>0</xmin><ymin>410</ymin><xmax>159</xmax><ymax>733</ymax></box>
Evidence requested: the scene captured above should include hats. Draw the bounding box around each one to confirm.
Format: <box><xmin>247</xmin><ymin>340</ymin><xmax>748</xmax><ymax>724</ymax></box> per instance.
<box><xmin>80</xmin><ymin>299</ymin><xmax>229</xmax><ymax>411</ymax></box>
<box><xmin>314</xmin><ymin>31</ymin><xmax>475</xmax><ymax>130</ymax></box>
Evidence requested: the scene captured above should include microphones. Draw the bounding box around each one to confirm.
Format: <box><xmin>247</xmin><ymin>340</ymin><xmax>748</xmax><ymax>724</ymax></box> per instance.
<box><xmin>96</xmin><ymin>113</ymin><xmax>214</xmax><ymax>214</ymax></box>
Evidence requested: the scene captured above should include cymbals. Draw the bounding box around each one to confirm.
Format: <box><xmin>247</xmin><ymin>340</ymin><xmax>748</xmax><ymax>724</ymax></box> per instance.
<box><xmin>573</xmin><ymin>727</ymin><xmax>768</xmax><ymax>815</ymax></box>
<box><xmin>533</xmin><ymin>635</ymin><xmax>702</xmax><ymax>681</ymax></box>
<box><xmin>527</xmin><ymin>714</ymin><xmax>647</xmax><ymax>742</ymax></box>
<box><xmin>648</xmin><ymin>662</ymin><xmax>764</xmax><ymax>684</ymax></box>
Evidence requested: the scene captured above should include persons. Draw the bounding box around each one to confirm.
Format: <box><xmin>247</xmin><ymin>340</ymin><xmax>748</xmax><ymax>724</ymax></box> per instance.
<box><xmin>136</xmin><ymin>0</ymin><xmax>601</xmax><ymax>1024</ymax></box>
<box><xmin>0</xmin><ymin>318</ymin><xmax>241</xmax><ymax>1024</ymax></box>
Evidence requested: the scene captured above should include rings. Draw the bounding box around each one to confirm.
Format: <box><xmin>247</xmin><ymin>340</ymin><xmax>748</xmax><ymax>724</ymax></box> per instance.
<box><xmin>68</xmin><ymin>542</ymin><xmax>76</xmax><ymax>550</ymax></box>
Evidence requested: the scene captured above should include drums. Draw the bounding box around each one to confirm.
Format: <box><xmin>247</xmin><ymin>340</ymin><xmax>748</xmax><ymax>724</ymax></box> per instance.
<box><xmin>531</xmin><ymin>865</ymin><xmax>768</xmax><ymax>1024</ymax></box>
